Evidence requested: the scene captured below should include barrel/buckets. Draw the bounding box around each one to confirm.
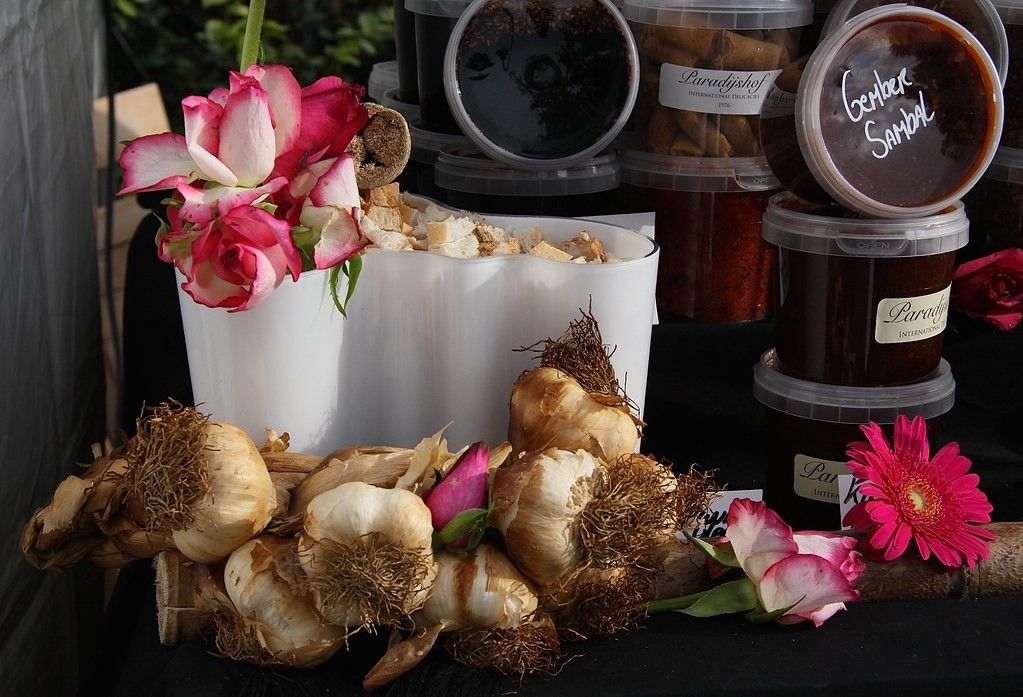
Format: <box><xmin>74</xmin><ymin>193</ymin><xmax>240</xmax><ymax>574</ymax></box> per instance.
<box><xmin>363</xmin><ymin>0</ymin><xmax>1023</xmax><ymax>541</ymax></box>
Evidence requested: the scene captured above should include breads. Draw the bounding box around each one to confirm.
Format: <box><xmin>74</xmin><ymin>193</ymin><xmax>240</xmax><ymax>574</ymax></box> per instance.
<box><xmin>355</xmin><ymin>181</ymin><xmax>622</xmax><ymax>262</ymax></box>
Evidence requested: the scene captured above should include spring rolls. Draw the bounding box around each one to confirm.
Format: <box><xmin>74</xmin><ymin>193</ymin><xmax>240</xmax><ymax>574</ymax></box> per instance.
<box><xmin>637</xmin><ymin>12</ymin><xmax>814</xmax><ymax>161</ymax></box>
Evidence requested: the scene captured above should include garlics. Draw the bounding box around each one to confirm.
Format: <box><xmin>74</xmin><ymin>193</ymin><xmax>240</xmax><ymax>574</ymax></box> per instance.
<box><xmin>107</xmin><ymin>287</ymin><xmax>728</xmax><ymax>680</ymax></box>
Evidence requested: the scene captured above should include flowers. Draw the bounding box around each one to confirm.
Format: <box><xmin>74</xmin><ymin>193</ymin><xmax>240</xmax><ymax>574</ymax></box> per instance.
<box><xmin>955</xmin><ymin>246</ymin><xmax>1023</xmax><ymax>333</ymax></box>
<box><xmin>616</xmin><ymin>499</ymin><xmax>863</xmax><ymax>627</ymax></box>
<box><xmin>838</xmin><ymin>412</ymin><xmax>995</xmax><ymax>580</ymax></box>
<box><xmin>116</xmin><ymin>0</ymin><xmax>374</xmax><ymax>320</ymax></box>
<box><xmin>425</xmin><ymin>442</ymin><xmax>496</xmax><ymax>558</ymax></box>
